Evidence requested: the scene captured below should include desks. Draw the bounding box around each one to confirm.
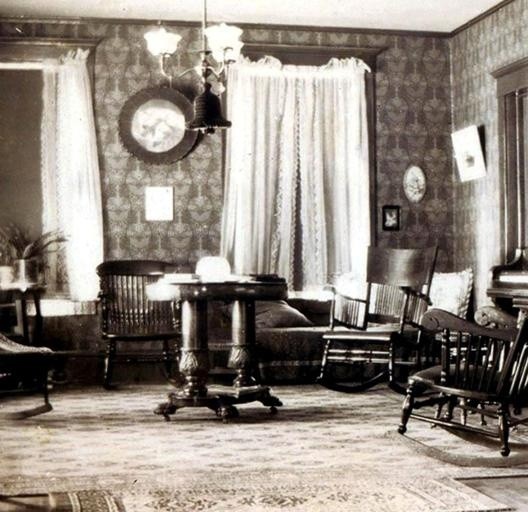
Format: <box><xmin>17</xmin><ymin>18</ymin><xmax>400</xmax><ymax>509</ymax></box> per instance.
<box><xmin>0</xmin><ymin>285</ymin><xmax>48</xmax><ymax>347</ymax></box>
<box><xmin>144</xmin><ymin>274</ymin><xmax>291</xmax><ymax>426</ymax></box>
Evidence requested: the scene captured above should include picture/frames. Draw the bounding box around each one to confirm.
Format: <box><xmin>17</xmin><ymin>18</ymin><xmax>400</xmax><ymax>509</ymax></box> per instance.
<box><xmin>117</xmin><ymin>83</ymin><xmax>198</xmax><ymax>166</ymax></box>
<box><xmin>380</xmin><ymin>204</ymin><xmax>402</xmax><ymax>231</ymax></box>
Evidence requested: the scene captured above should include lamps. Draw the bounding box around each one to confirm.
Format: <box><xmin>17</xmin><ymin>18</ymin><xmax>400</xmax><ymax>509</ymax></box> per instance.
<box><xmin>142</xmin><ymin>0</ymin><xmax>243</xmax><ymax>137</ymax></box>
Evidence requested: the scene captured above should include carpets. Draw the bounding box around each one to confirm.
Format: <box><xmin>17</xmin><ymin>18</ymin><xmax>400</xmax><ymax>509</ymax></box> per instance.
<box><xmin>1</xmin><ymin>376</ymin><xmax>526</xmax><ymax>498</ymax></box>
<box><xmin>49</xmin><ymin>480</ymin><xmax>515</xmax><ymax>512</ymax></box>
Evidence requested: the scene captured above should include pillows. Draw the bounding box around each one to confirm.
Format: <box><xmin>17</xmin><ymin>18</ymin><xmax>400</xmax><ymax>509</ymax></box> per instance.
<box><xmin>217</xmin><ymin>297</ymin><xmax>316</xmax><ymax>331</ymax></box>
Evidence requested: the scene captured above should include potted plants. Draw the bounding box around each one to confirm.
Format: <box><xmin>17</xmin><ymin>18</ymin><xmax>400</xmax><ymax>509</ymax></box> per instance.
<box><xmin>0</xmin><ymin>217</ymin><xmax>69</xmax><ymax>286</ymax></box>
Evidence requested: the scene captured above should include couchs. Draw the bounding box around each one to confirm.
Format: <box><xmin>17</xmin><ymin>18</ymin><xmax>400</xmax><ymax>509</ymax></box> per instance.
<box><xmin>206</xmin><ymin>267</ymin><xmax>475</xmax><ymax>392</ymax></box>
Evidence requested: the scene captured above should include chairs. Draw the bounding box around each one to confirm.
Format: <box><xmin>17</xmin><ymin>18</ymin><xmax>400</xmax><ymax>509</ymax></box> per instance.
<box><xmin>315</xmin><ymin>240</ymin><xmax>439</xmax><ymax>391</ymax></box>
<box><xmin>394</xmin><ymin>293</ymin><xmax>528</xmax><ymax>456</ymax></box>
<box><xmin>1</xmin><ymin>331</ymin><xmax>59</xmax><ymax>408</ymax></box>
<box><xmin>89</xmin><ymin>257</ymin><xmax>188</xmax><ymax>390</ymax></box>
